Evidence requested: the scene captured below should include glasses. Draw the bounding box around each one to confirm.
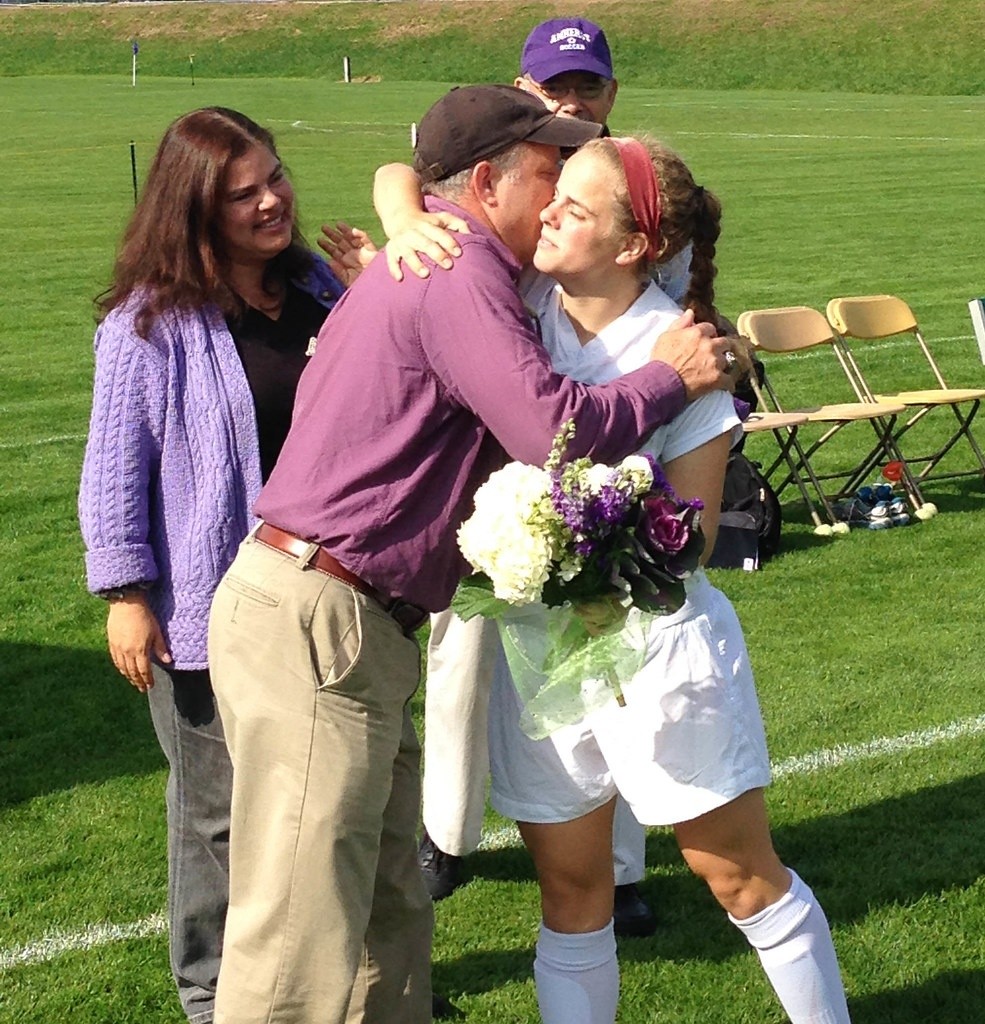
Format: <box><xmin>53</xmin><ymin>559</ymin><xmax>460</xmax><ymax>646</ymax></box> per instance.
<box><xmin>526</xmin><ymin>73</ymin><xmax>609</xmax><ymax>99</ymax></box>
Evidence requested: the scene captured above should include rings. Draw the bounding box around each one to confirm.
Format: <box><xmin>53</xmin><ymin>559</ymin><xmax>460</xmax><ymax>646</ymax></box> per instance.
<box><xmin>724</xmin><ymin>351</ymin><xmax>738</xmax><ymax>370</ymax></box>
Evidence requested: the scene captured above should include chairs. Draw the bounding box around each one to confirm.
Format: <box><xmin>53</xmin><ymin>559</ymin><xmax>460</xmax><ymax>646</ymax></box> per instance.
<box><xmin>825</xmin><ymin>295</ymin><xmax>985</xmax><ymax>521</ymax></box>
<box><xmin>736</xmin><ymin>306</ymin><xmax>934</xmax><ymax>534</ymax></box>
<box><xmin>735</xmin><ymin>334</ymin><xmax>836</xmax><ymax>535</ymax></box>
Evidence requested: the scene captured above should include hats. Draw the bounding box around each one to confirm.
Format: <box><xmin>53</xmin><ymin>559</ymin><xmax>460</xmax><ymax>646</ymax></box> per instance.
<box><xmin>522</xmin><ymin>18</ymin><xmax>611</xmax><ymax>86</ymax></box>
<box><xmin>409</xmin><ymin>83</ymin><xmax>605</xmax><ymax>187</ymax></box>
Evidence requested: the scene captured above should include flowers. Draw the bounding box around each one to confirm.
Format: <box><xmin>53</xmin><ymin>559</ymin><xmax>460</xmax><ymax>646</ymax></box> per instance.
<box><xmin>452</xmin><ymin>413</ymin><xmax>712</xmax><ymax>622</ymax></box>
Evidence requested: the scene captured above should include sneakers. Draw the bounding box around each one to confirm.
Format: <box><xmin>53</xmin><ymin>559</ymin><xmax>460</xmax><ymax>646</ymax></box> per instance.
<box><xmin>827</xmin><ymin>484</ymin><xmax>893</xmax><ymax>529</ymax></box>
<box><xmin>871</xmin><ymin>483</ymin><xmax>910</xmax><ymax>523</ymax></box>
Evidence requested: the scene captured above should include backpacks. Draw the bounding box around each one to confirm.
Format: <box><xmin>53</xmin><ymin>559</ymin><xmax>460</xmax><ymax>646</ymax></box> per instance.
<box><xmin>708</xmin><ymin>450</ymin><xmax>782</xmax><ymax>569</ymax></box>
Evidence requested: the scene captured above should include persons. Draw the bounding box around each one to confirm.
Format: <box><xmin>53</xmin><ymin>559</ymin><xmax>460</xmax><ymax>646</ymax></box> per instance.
<box><xmin>210</xmin><ymin>80</ymin><xmax>759</xmax><ymax>1024</ymax></box>
<box><xmin>371</xmin><ymin>136</ymin><xmax>851</xmax><ymax>1024</ymax></box>
<box><xmin>75</xmin><ymin>107</ymin><xmax>382</xmax><ymax>1024</ymax></box>
<box><xmin>416</xmin><ymin>16</ymin><xmax>658</xmax><ymax>936</ymax></box>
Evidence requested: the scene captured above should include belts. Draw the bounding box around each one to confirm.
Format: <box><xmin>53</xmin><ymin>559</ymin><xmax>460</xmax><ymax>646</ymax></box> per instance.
<box><xmin>253</xmin><ymin>519</ymin><xmax>429</xmax><ymax>634</ymax></box>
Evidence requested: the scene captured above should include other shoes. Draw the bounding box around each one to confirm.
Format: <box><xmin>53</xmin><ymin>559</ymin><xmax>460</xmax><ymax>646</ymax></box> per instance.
<box><xmin>612</xmin><ymin>886</ymin><xmax>653</xmax><ymax>937</ymax></box>
<box><xmin>417</xmin><ymin>836</ymin><xmax>462</xmax><ymax>901</ymax></box>
